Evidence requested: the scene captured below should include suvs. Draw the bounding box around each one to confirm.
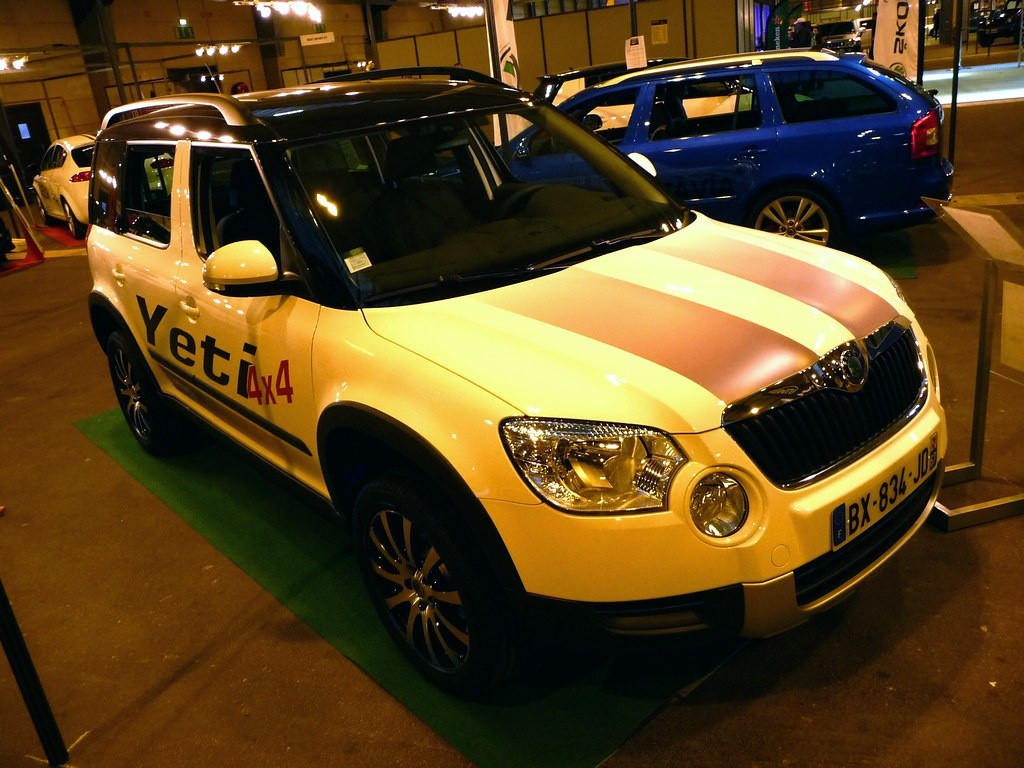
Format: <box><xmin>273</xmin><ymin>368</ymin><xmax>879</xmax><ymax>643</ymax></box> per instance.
<box><xmin>493</xmin><ymin>45</ymin><xmax>955</xmax><ymax>250</ymax></box>
<box><xmin>977</xmin><ymin>9</ymin><xmax>1023</xmax><ymax>47</ymax></box>
<box><xmin>813</xmin><ymin>21</ymin><xmax>866</xmax><ymax>51</ymax></box>
<box><xmin>926</xmin><ymin>16</ymin><xmax>942</xmax><ymax>39</ymax></box>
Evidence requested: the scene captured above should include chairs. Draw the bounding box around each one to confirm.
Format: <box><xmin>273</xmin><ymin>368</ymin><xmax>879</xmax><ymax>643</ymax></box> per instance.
<box><xmin>649</xmin><ymin>103</ymin><xmax>675</xmax><ymax>140</ymax></box>
<box><xmin>371</xmin><ymin>134</ymin><xmax>481</xmax><ymax>257</ymax></box>
<box><xmin>213</xmin><ymin>156</ymin><xmax>279</xmax><ymax>251</ymax></box>
<box><xmin>292</xmin><ymin>141</ymin><xmax>371</xmax><ymax>253</ymax></box>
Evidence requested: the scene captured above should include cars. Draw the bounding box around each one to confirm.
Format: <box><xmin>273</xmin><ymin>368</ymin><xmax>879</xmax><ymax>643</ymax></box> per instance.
<box><xmin>32</xmin><ymin>133</ymin><xmax>97</xmax><ymax>241</ymax></box>
<box><xmin>968</xmin><ymin>10</ymin><xmax>992</xmax><ymax>34</ymax></box>
<box><xmin>87</xmin><ymin>64</ymin><xmax>948</xmax><ymax>688</ymax></box>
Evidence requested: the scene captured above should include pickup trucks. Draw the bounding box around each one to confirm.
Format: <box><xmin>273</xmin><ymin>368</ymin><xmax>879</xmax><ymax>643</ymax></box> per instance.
<box><xmin>856</xmin><ymin>17</ymin><xmax>875</xmax><ymax>49</ymax></box>
